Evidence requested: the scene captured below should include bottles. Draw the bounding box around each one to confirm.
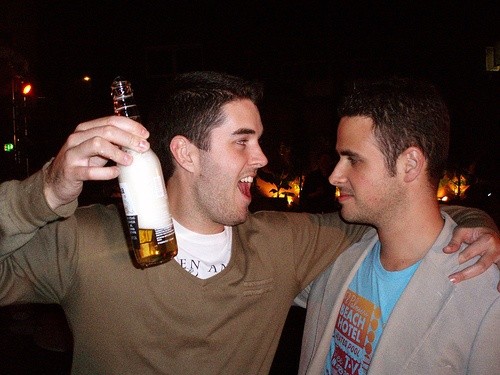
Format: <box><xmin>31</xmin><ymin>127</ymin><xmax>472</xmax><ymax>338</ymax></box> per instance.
<box><xmin>110</xmin><ymin>80</ymin><xmax>178</xmax><ymax>267</ymax></box>
